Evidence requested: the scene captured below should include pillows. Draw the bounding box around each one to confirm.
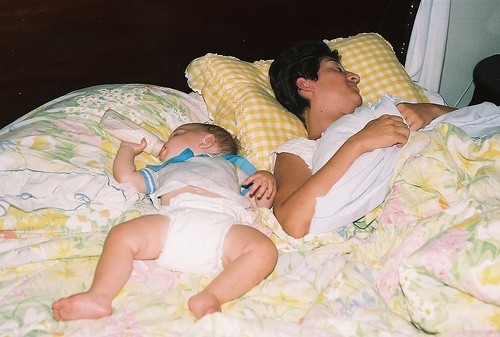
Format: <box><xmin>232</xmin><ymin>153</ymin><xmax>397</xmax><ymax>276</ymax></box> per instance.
<box><xmin>184</xmin><ymin>33</ymin><xmax>427</xmax><ymax>172</ymax></box>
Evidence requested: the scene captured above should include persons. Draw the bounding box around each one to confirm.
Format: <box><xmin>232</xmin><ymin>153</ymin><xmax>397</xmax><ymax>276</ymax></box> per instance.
<box><xmin>269</xmin><ymin>41</ymin><xmax>459</xmax><ymax>239</ymax></box>
<box><xmin>51</xmin><ymin>122</ymin><xmax>278</xmax><ymax>321</ymax></box>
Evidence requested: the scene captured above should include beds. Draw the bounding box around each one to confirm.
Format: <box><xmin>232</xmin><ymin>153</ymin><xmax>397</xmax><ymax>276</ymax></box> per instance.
<box><xmin>0</xmin><ymin>0</ymin><xmax>500</xmax><ymax>337</ymax></box>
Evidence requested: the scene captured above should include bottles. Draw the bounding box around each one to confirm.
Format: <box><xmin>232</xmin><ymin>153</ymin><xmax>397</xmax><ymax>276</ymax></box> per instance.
<box><xmin>99</xmin><ymin>109</ymin><xmax>166</xmax><ymax>157</ymax></box>
